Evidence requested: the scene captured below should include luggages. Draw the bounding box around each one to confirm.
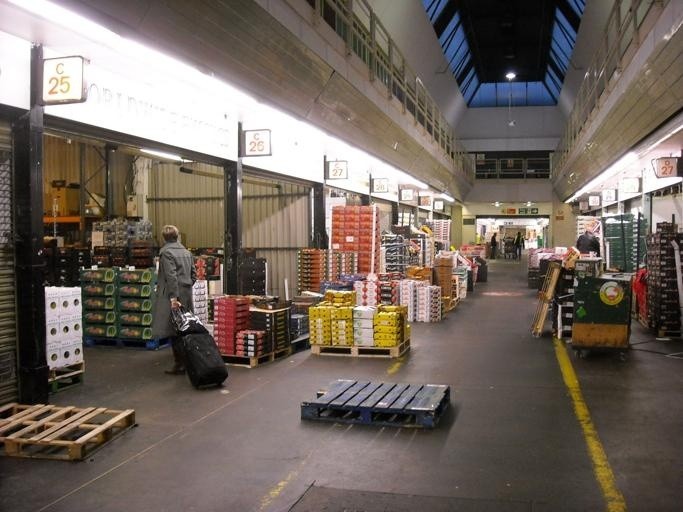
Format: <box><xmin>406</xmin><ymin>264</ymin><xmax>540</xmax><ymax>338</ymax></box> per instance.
<box><xmin>171</xmin><ymin>307</ymin><xmax>229</xmax><ymax>388</ymax></box>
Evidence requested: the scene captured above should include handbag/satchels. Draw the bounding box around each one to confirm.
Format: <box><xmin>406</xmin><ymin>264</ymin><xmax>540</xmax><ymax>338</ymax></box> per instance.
<box><xmin>577</xmin><ymin>231</ymin><xmax>600</xmax><ymax>257</ymax></box>
<box><xmin>172</xmin><ymin>306</ymin><xmax>209</xmax><ymax>337</ymax></box>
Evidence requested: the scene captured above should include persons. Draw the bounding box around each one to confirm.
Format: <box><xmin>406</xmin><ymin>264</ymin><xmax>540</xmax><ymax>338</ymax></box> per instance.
<box><xmin>150</xmin><ymin>225</ymin><xmax>197</xmax><ymax>376</ymax></box>
<box><xmin>513</xmin><ymin>231</ymin><xmax>524</xmax><ymax>260</ymax></box>
<box><xmin>576</xmin><ymin>226</ymin><xmax>600</xmax><ymax>269</ymax></box>
<box><xmin>491</xmin><ymin>232</ymin><xmax>498</xmax><ymax>259</ymax></box>
<box><xmin>503</xmin><ymin>233</ymin><xmax>515</xmax><ymax>242</ymax></box>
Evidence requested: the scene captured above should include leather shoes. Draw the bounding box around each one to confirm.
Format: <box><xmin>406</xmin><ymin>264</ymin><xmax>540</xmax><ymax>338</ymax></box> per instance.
<box><xmin>164</xmin><ymin>366</ymin><xmax>186</xmax><ymax>375</ymax></box>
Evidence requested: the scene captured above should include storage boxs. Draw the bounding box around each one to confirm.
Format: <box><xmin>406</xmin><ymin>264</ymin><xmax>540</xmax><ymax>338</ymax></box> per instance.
<box><xmin>80</xmin><ymin>245</ymin><xmax>291</xmax><ymax>362</ymax></box>
<box><xmin>309</xmin><ymin>204</ymin><xmax>455</xmax><ymax>349</ymax></box>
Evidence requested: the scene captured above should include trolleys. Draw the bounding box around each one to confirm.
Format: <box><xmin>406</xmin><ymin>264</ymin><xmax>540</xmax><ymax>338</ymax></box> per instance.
<box><xmin>502</xmin><ymin>236</ymin><xmax>518</xmax><ymax>259</ymax></box>
